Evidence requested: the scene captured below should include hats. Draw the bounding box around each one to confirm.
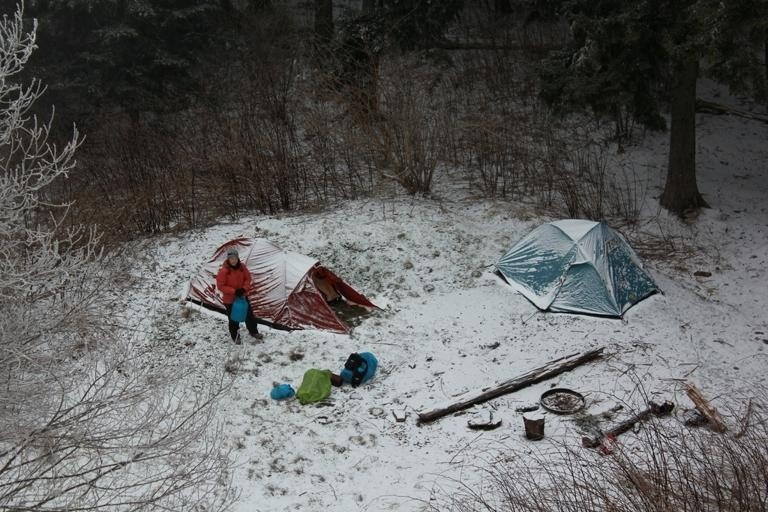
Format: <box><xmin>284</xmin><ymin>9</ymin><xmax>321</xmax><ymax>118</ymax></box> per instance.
<box><xmin>226</xmin><ymin>249</ymin><xmax>239</xmax><ymax>258</ymax></box>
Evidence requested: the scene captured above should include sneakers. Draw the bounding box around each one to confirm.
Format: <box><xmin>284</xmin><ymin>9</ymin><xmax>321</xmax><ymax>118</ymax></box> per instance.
<box><xmin>251</xmin><ymin>332</ymin><xmax>263</xmax><ymax>339</ymax></box>
<box><xmin>233</xmin><ymin>336</ymin><xmax>241</xmax><ymax>345</ymax></box>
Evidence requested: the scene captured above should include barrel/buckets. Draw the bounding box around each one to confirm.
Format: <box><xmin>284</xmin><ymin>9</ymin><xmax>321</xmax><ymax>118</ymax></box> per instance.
<box><xmin>523</xmin><ymin>412</ymin><xmax>545</xmax><ymax>441</ymax></box>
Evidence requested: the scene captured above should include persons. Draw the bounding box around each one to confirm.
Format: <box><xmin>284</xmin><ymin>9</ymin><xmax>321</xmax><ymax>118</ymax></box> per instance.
<box><xmin>217</xmin><ymin>250</ymin><xmax>262</xmax><ymax>343</ymax></box>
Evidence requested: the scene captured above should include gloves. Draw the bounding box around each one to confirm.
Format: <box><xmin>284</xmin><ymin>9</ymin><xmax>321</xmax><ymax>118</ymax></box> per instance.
<box><xmin>235</xmin><ymin>288</ymin><xmax>245</xmax><ymax>296</ymax></box>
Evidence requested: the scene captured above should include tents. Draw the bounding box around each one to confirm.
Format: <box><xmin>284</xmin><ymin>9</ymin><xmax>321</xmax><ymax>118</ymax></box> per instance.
<box><xmin>186</xmin><ymin>237</ymin><xmax>383</xmax><ymax>334</ymax></box>
<box><xmin>493</xmin><ymin>219</ymin><xmax>664</xmax><ymax>319</ymax></box>
